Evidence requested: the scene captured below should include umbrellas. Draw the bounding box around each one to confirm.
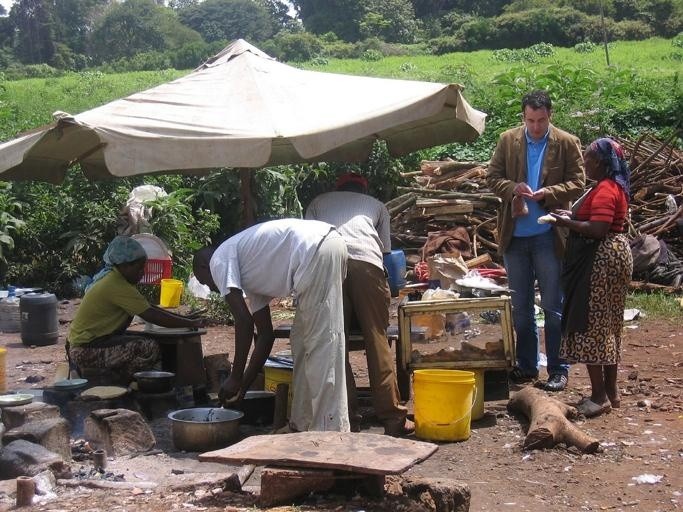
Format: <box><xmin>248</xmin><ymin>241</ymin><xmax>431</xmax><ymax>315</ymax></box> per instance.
<box><xmin>1</xmin><ymin>36</ymin><xmax>489</xmax><ymax>228</ymax></box>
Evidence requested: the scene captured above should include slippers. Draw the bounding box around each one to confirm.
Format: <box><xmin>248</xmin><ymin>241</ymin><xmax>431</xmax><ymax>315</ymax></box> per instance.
<box><xmin>575</xmin><ymin>400</ymin><xmax>620</xmax><ymax>416</ymax></box>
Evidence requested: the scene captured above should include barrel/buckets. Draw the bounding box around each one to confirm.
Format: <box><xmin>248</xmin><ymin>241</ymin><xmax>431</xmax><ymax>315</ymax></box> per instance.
<box><xmin>19</xmin><ymin>293</ymin><xmax>57</xmax><ymax>345</ymax></box>
<box><xmin>412</xmin><ymin>369</ymin><xmax>477</xmax><ymax>441</ymax></box>
<box><xmin>204</xmin><ymin>353</ymin><xmax>232</xmax><ymax>393</ymax></box>
<box><xmin>383</xmin><ymin>249</ymin><xmax>406</xmax><ymax>297</ymax></box>
<box><xmin>0</xmin><ymin>346</ymin><xmax>8</xmax><ymax>393</ymax></box>
<box><xmin>0</xmin><ymin>286</ymin><xmax>20</xmax><ymax>332</ymax></box>
<box><xmin>468</xmin><ymin>369</ymin><xmax>486</xmax><ymax>421</ymax></box>
<box><xmin>263</xmin><ymin>355</ymin><xmax>294</xmax><ymax>419</ymax></box>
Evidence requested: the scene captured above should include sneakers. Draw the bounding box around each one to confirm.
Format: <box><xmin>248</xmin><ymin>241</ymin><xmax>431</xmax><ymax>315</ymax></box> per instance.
<box><xmin>394</xmin><ymin>419</ymin><xmax>414</xmax><ymax>436</ymax></box>
<box><xmin>544</xmin><ymin>374</ymin><xmax>568</xmax><ymax>391</ymax></box>
<box><xmin>506</xmin><ymin>367</ymin><xmax>538</xmax><ymax>384</ymax></box>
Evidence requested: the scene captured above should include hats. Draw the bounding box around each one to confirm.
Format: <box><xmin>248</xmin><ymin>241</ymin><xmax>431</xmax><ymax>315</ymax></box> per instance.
<box><xmin>335</xmin><ymin>173</ymin><xmax>366</xmax><ymax>194</ymax></box>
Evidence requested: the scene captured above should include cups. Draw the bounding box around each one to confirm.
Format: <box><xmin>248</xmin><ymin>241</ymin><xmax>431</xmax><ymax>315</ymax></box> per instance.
<box><xmin>16</xmin><ymin>476</ymin><xmax>32</xmax><ymax>506</ymax></box>
<box><xmin>93</xmin><ymin>449</ymin><xmax>106</xmax><ymax>468</ymax></box>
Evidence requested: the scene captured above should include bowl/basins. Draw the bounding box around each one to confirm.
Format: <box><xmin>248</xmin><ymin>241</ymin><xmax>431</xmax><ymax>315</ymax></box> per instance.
<box><xmin>207</xmin><ymin>391</ymin><xmax>275</xmax><ymax>424</ymax></box>
<box><xmin>132</xmin><ymin>370</ymin><xmax>176</xmax><ymax>392</ymax></box>
<box><xmin>166</xmin><ymin>407</ymin><xmax>244</xmax><ymax>452</ymax></box>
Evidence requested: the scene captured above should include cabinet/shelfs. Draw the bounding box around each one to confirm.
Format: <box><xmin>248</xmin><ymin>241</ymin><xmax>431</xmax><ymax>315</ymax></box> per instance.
<box><xmin>399</xmin><ymin>295</ymin><xmax>516</xmax><ymax>371</ymax></box>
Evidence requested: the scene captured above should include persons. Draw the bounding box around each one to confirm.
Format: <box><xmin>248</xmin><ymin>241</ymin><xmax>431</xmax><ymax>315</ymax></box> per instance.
<box><xmin>484</xmin><ymin>88</ymin><xmax>586</xmax><ymax>387</ymax></box>
<box><xmin>304</xmin><ymin>171</ymin><xmax>415</xmax><ymax>439</ymax></box>
<box><xmin>536</xmin><ymin>137</ymin><xmax>634</xmax><ymax>416</ymax></box>
<box><xmin>192</xmin><ymin>217</ymin><xmax>353</xmax><ymax>434</ymax></box>
<box><xmin>68</xmin><ymin>236</ymin><xmax>221</xmax><ymax>386</ymax></box>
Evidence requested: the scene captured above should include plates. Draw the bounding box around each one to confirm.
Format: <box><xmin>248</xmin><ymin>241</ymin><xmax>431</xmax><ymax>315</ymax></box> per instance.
<box><xmin>0</xmin><ymin>394</ymin><xmax>33</xmax><ymax>405</ymax></box>
<box><xmin>54</xmin><ymin>378</ymin><xmax>88</xmax><ymax>390</ymax></box>
